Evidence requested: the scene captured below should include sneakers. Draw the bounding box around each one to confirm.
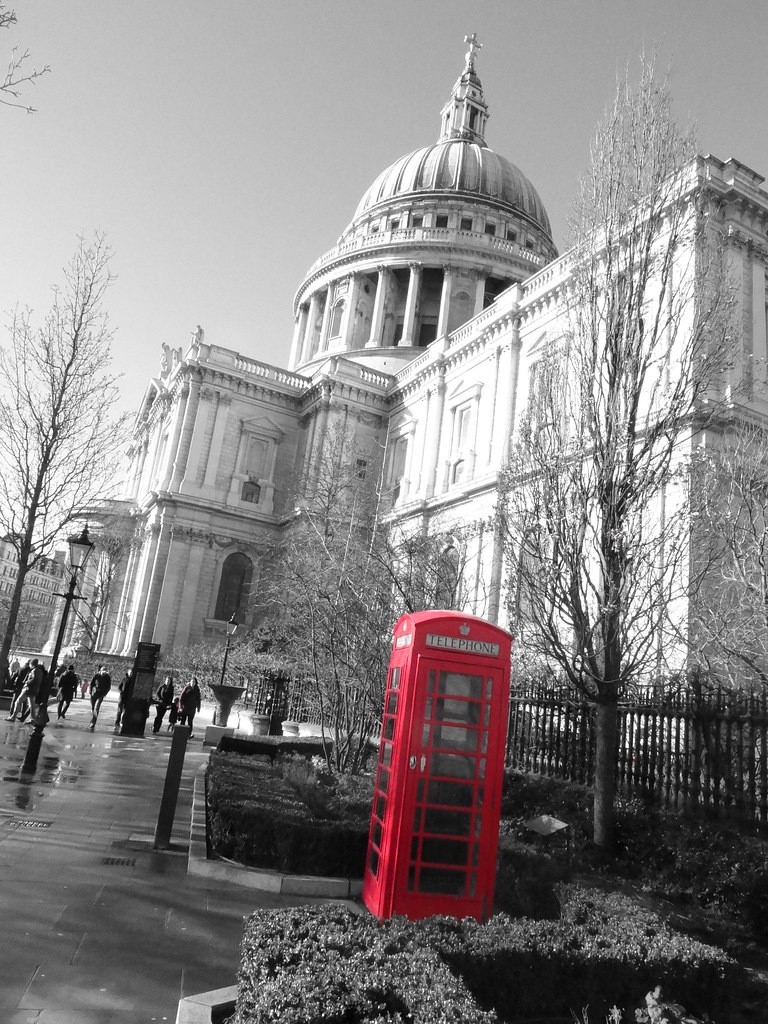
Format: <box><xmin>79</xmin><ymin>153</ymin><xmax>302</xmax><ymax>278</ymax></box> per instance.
<box><xmin>87</xmin><ymin>721</ymin><xmax>95</xmax><ymax>729</ymax></box>
<box><xmin>114</xmin><ymin>724</ymin><xmax>120</xmax><ymax>729</ymax></box>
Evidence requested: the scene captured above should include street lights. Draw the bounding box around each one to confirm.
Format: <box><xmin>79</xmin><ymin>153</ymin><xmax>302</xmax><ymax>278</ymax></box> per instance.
<box><xmin>220</xmin><ymin>613</ymin><xmax>239</xmax><ymax>686</ymax></box>
<box><xmin>20</xmin><ymin>522</ymin><xmax>96</xmax><ymax>774</ymax></box>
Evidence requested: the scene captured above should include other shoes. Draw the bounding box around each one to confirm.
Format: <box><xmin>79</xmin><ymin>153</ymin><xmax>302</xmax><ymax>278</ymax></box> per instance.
<box><xmin>81</xmin><ymin>695</ymin><xmax>84</xmax><ymax>699</ymax></box>
<box><xmin>4</xmin><ymin>714</ymin><xmax>35</xmax><ymax>725</ymax></box>
<box><xmin>152</xmin><ymin>730</ymin><xmax>159</xmax><ymax>735</ymax></box>
<box><xmin>189</xmin><ymin>734</ymin><xmax>194</xmax><ymax>740</ymax></box>
<box><xmin>57</xmin><ymin>713</ymin><xmax>66</xmax><ymax>720</ymax></box>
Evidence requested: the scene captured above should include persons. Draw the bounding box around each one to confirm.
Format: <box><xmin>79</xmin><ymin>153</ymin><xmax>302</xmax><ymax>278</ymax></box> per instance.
<box><xmin>56</xmin><ymin>664</ymin><xmax>67</xmax><ymax>677</ymax></box>
<box><xmin>152</xmin><ymin>676</ymin><xmax>174</xmax><ymax>734</ymax></box>
<box><xmin>10</xmin><ymin>660</ymin><xmax>31</xmax><ymax>716</ymax></box>
<box><xmin>167</xmin><ymin>698</ymin><xmax>180</xmax><ymax>733</ymax></box>
<box><xmin>80</xmin><ymin>679</ymin><xmax>89</xmax><ymax>699</ymax></box>
<box><xmin>58</xmin><ymin>665</ymin><xmax>77</xmax><ymax>719</ymax></box>
<box><xmin>88</xmin><ymin>667</ymin><xmax>112</xmax><ymax>728</ymax></box>
<box><xmin>113</xmin><ymin>668</ymin><xmax>134</xmax><ymax>730</ymax></box>
<box><xmin>4</xmin><ymin>659</ymin><xmax>43</xmax><ymax>724</ymax></box>
<box><xmin>35</xmin><ymin>665</ymin><xmax>49</xmax><ymax>724</ymax></box>
<box><xmin>190</xmin><ymin>326</ymin><xmax>203</xmax><ymax>341</ymax></box>
<box><xmin>262</xmin><ymin>692</ymin><xmax>273</xmax><ymax>715</ymax></box>
<box><xmin>75</xmin><ymin>674</ymin><xmax>82</xmax><ymax>698</ymax></box>
<box><xmin>10</xmin><ymin>658</ymin><xmax>20</xmax><ymax>676</ymax></box>
<box><xmin>180</xmin><ymin>677</ymin><xmax>201</xmax><ymax>738</ymax></box>
<box><xmin>143</xmin><ymin>698</ymin><xmax>160</xmax><ymax>736</ymax></box>
